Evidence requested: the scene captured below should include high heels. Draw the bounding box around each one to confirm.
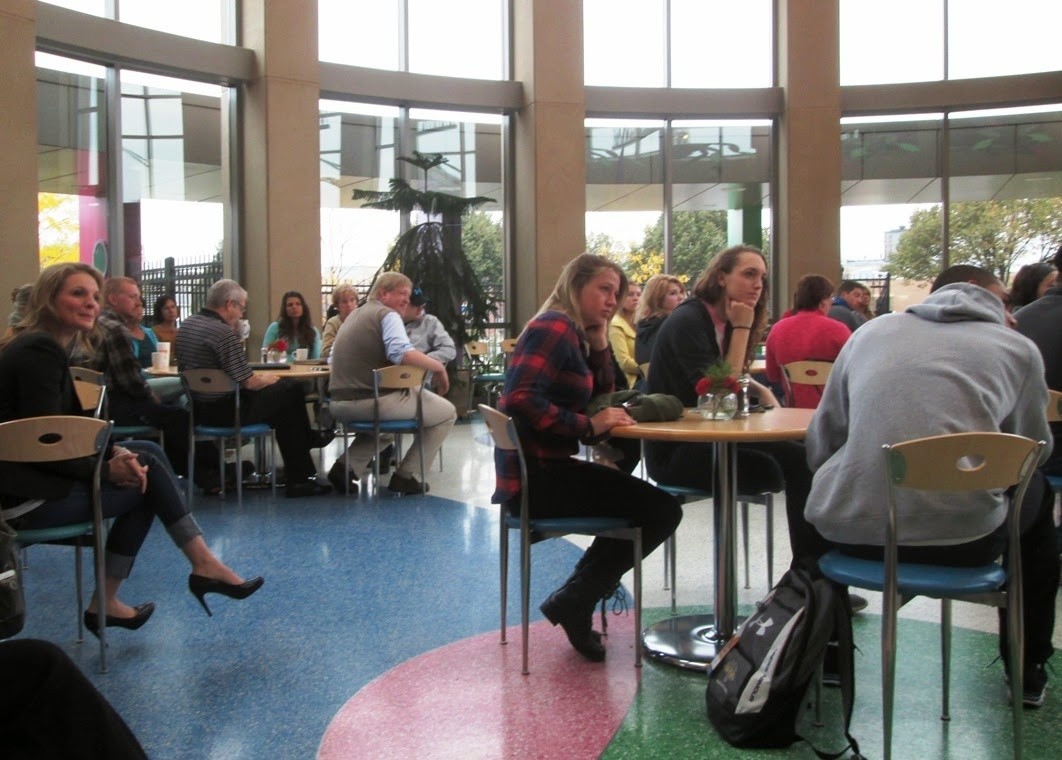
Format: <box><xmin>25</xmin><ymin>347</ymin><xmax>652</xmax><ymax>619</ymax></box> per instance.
<box><xmin>188</xmin><ymin>572</ymin><xmax>264</xmax><ymax>618</ymax></box>
<box><xmin>85</xmin><ymin>600</ymin><xmax>157</xmax><ymax>646</ymax></box>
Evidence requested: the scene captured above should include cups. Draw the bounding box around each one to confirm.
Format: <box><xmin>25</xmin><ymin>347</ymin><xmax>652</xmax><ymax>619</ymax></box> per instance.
<box><xmin>292</xmin><ymin>348</ymin><xmax>308</xmax><ymax>361</ymax></box>
<box><xmin>152</xmin><ymin>341</ymin><xmax>171</xmax><ymax>371</ymax></box>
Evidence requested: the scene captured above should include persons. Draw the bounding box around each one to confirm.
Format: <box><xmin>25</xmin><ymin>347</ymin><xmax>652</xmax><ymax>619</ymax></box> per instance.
<box><xmin>489</xmin><ymin>242</ymin><xmax>1062</xmax><ymax>712</ymax></box>
<box><xmin>0</xmin><ymin>262</ymin><xmax>266</xmax><ymax>648</ymax></box>
<box><xmin>92</xmin><ymin>272</ymin><xmax>457</xmax><ymax>501</ymax></box>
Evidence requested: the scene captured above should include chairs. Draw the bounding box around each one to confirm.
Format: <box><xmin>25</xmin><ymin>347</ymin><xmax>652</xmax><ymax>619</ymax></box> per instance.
<box><xmin>779</xmin><ymin>358</ymin><xmax>833</xmax><ymax>409</ymax></box>
<box><xmin>72</xmin><ymin>381</ymin><xmax>105</xmax><ymax>420</ymax></box>
<box><xmin>68</xmin><ymin>366</ymin><xmax>164</xmax><ymax>452</ymax></box>
<box><xmin>0</xmin><ymin>416</ymin><xmax>116</xmax><ymax>673</ymax></box>
<box><xmin>342</xmin><ymin>365</ymin><xmax>432</xmax><ymax>497</ymax></box>
<box><xmin>477</xmin><ymin>402</ymin><xmax>646</xmax><ymax>675</ymax></box>
<box><xmin>463</xmin><ymin>342</ymin><xmax>508</xmax><ymax>419</ymax></box>
<box><xmin>502</xmin><ymin>338</ymin><xmax>517</xmax><ymax>366</ymax></box>
<box><xmin>817</xmin><ymin>431</ymin><xmax>1047</xmax><ymax>760</ymax></box>
<box><xmin>179</xmin><ymin>368</ymin><xmax>277</xmax><ymax>498</ymax></box>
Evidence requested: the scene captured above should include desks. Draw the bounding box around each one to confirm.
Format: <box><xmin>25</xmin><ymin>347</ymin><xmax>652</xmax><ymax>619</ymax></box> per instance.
<box><xmin>610</xmin><ymin>407</ymin><xmax>819</xmax><ymax>673</ymax></box>
<box><xmin>748</xmin><ymin>359</ymin><xmax>768</xmax><ymax>391</ymax></box>
<box><xmin>143</xmin><ymin>363</ymin><xmax>331</xmax><ymax>489</ymax></box>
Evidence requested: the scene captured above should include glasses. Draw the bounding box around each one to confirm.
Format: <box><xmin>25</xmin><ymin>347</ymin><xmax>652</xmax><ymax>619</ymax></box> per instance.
<box><xmin>235</xmin><ymin>301</ymin><xmax>249</xmax><ymax>318</ymax></box>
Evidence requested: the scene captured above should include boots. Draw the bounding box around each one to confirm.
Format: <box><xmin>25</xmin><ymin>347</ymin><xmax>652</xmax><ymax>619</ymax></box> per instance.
<box><xmin>543</xmin><ymin>563</ymin><xmax>620</xmax><ymax>664</ymax></box>
<box><xmin>549</xmin><ymin>548</ymin><xmax>592</xmax><ymax>597</ymax></box>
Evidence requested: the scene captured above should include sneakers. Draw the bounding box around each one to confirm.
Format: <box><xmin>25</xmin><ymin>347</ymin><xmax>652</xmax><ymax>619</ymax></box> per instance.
<box><xmin>1004</xmin><ymin>659</ymin><xmax>1048</xmax><ymax>706</ymax></box>
<box><xmin>208</xmin><ymin>460</ymin><xmax>255</xmax><ymax>492</ymax></box>
<box><xmin>823</xmin><ymin>639</ymin><xmax>843</xmax><ymax>684</ymax></box>
<box><xmin>388</xmin><ymin>472</ymin><xmax>429</xmax><ymax>493</ymax></box>
<box><xmin>329</xmin><ymin>462</ymin><xmax>358</xmax><ymax>497</ymax></box>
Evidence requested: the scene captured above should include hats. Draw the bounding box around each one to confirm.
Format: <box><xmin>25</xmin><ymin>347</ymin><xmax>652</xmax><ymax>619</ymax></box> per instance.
<box><xmin>409</xmin><ymin>285</ymin><xmax>431</xmax><ymax>307</ymax></box>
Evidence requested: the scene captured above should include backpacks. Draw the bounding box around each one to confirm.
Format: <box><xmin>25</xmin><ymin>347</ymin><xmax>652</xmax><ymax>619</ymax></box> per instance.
<box><xmin>706</xmin><ymin>559</ymin><xmax>864</xmax><ymax>759</ymax></box>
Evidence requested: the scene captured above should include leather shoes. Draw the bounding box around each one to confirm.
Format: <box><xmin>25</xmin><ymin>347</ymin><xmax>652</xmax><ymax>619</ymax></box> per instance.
<box><xmin>373</xmin><ymin>456</ymin><xmax>390</xmax><ymax>475</ymax></box>
<box><xmin>288</xmin><ymin>479</ymin><xmax>332</xmax><ymax>497</ymax></box>
